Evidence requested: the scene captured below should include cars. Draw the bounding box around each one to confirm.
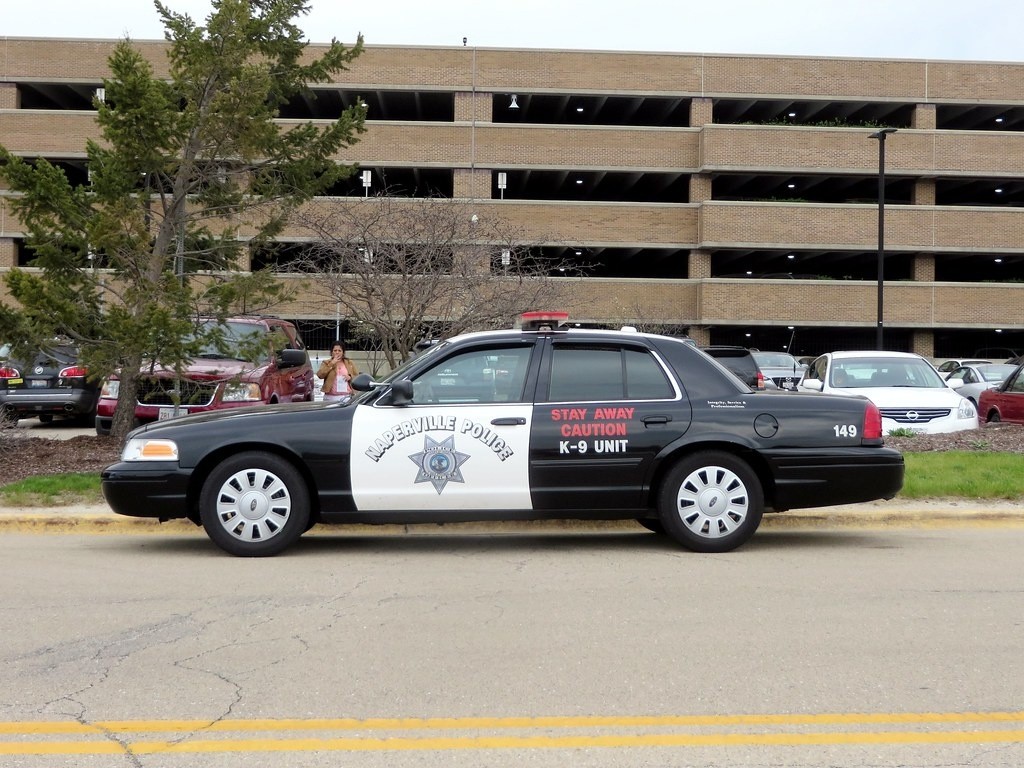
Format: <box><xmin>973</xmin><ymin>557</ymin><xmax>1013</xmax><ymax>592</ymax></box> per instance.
<box><xmin>934</xmin><ymin>360</ymin><xmax>991</xmax><ymax>379</ymax></box>
<box><xmin>751</xmin><ymin>351</ymin><xmax>806</xmax><ymax>392</ymax></box>
<box><xmin>399</xmin><ymin>341</ymin><xmax>500</xmax><ymax>404</ymax></box>
<box><xmin>943</xmin><ymin>364</ymin><xmax>1019</xmax><ymax>412</ymax></box>
<box><xmin>695</xmin><ymin>345</ymin><xmax>766</xmax><ymax>394</ymax></box>
<box><xmin>98</xmin><ymin>313</ymin><xmax>910</xmax><ymax>557</ymax></box>
<box><xmin>307</xmin><ymin>355</ymin><xmax>332</xmax><ymax>401</ymax></box>
<box><xmin>976</xmin><ymin>362</ymin><xmax>1024</xmax><ymax>426</ymax></box>
<box><xmin>0</xmin><ymin>336</ymin><xmax>106</xmax><ymax>429</ymax></box>
<box><xmin>794</xmin><ymin>351</ymin><xmax>979</xmax><ymax>436</ymax></box>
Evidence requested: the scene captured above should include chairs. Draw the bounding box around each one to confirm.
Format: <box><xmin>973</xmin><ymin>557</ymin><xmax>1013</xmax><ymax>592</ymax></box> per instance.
<box><xmin>834</xmin><ymin>366</ymin><xmax>906</xmax><ymax>387</ymax></box>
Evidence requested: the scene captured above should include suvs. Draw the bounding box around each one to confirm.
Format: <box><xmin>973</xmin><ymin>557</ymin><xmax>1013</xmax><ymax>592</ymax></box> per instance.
<box><xmin>95</xmin><ymin>315</ymin><xmax>315</xmax><ymax>440</ymax></box>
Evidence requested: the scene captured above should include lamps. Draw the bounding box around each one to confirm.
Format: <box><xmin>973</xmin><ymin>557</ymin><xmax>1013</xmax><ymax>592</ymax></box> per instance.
<box><xmin>471</xmin><ymin>215</ymin><xmax>479</xmax><ymax>229</ymax></box>
<box><xmin>501</xmin><ymin>249</ymin><xmax>510</xmax><ymax>276</ymax></box>
<box><xmin>87</xmin><ymin>243</ymin><xmax>97</xmax><ymax>269</ymax></box>
<box><xmin>508</xmin><ymin>94</ymin><xmax>520</xmax><ymax>109</ymax></box>
<box><xmin>497</xmin><ymin>172</ymin><xmax>508</xmax><ymax>200</ymax></box>
<box><xmin>364</xmin><ymin>246</ymin><xmax>374</xmax><ymax>264</ymax></box>
<box><xmin>362</xmin><ymin>170</ymin><xmax>372</xmax><ymax>197</ymax></box>
<box><xmin>87</xmin><ymin>167</ymin><xmax>97</xmax><ymax>192</ymax></box>
<box><xmin>97</xmin><ymin>88</ymin><xmax>106</xmax><ymax>104</ymax></box>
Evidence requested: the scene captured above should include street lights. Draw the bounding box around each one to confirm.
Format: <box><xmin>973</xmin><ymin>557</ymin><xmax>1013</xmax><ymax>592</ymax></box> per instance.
<box><xmin>867</xmin><ymin>127</ymin><xmax>898</xmax><ymax>350</ymax></box>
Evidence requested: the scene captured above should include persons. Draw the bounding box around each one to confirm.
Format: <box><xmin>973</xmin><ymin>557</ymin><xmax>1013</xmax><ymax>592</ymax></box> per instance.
<box><xmin>317</xmin><ymin>341</ymin><xmax>359</xmax><ymax>401</ymax></box>
